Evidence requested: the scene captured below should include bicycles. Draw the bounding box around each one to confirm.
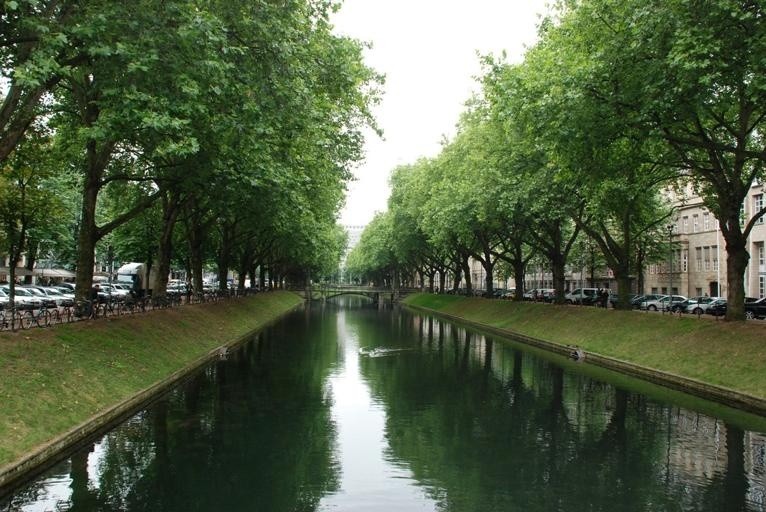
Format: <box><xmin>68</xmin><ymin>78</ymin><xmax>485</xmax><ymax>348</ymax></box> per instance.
<box><xmin>0</xmin><ymin>289</ymin><xmax>273</xmax><ymax>332</ymax></box>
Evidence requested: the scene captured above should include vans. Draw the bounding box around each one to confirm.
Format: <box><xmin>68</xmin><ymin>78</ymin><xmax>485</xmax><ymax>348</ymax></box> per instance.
<box><xmin>566</xmin><ymin>288</ymin><xmax>611</xmax><ymax>305</ymax></box>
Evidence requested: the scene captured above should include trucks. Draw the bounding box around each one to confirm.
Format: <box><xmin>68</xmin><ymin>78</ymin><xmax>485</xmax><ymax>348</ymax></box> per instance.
<box><xmin>118</xmin><ymin>263</ymin><xmax>157</xmax><ymax>302</ymax></box>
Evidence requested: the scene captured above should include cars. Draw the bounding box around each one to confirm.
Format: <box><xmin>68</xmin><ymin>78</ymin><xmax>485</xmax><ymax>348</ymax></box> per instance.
<box><xmin>166</xmin><ymin>279</ymin><xmax>274</xmax><ymax>297</ymax></box>
<box><xmin>0</xmin><ymin>283</ymin><xmax>133</xmax><ymax>311</ymax></box>
<box><xmin>611</xmin><ymin>294</ymin><xmax>766</xmax><ymax>320</ymax></box>
<box><xmin>447</xmin><ymin>288</ymin><xmax>555</xmax><ymax>303</ymax></box>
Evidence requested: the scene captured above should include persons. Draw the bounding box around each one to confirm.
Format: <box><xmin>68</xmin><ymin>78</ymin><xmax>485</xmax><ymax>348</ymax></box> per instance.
<box><xmin>595</xmin><ymin>288</ymin><xmax>609</xmax><ymax>308</ymax></box>
<box><xmin>93</xmin><ymin>285</ymin><xmax>100</xmax><ymax>307</ymax></box>
<box><xmin>533</xmin><ymin>288</ymin><xmax>538</xmax><ymax>303</ymax></box>
<box><xmin>126</xmin><ymin>290</ymin><xmax>135</xmax><ymax>301</ymax></box>
<box><xmin>705</xmin><ymin>293</ymin><xmax>707</xmax><ymax>296</ymax></box>
<box><xmin>541</xmin><ymin>290</ymin><xmax>544</xmax><ymax>303</ymax></box>
<box><xmin>185</xmin><ymin>280</ymin><xmax>193</xmax><ymax>302</ymax></box>
